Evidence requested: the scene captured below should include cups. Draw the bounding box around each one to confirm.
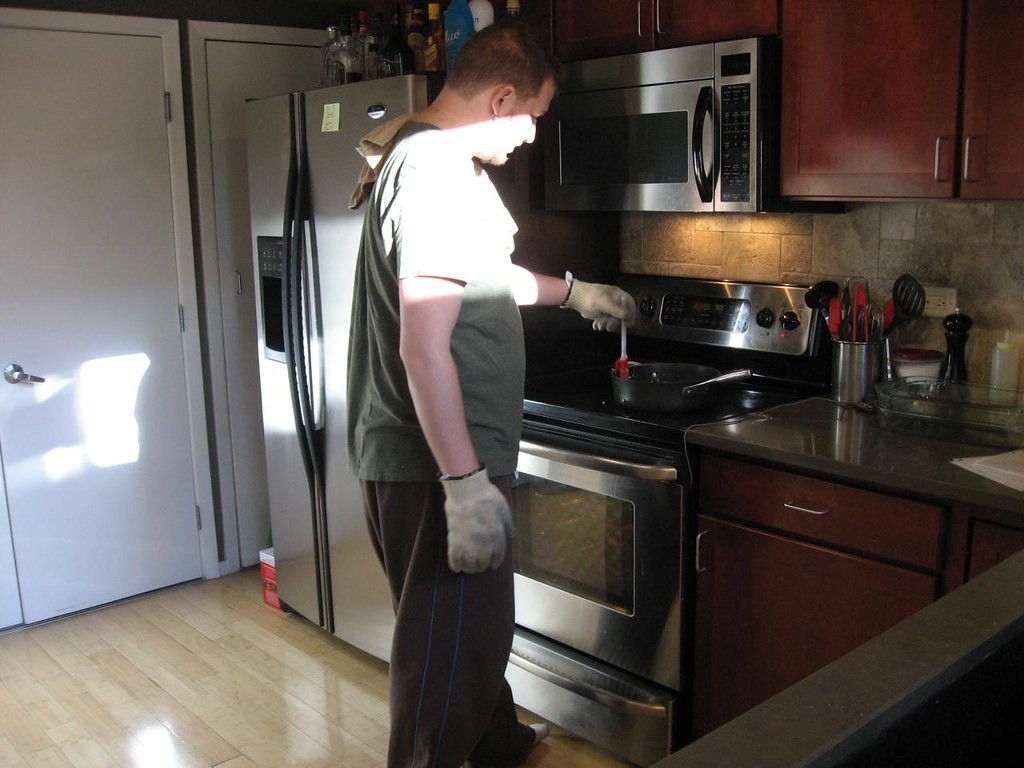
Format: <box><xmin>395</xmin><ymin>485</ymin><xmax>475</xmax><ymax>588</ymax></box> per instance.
<box><xmin>830</xmin><ymin>338</ymin><xmax>885</xmax><ymax>403</ymax></box>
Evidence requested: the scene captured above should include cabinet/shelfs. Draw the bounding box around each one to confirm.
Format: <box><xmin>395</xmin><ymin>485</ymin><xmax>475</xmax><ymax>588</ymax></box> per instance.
<box><xmin>779</xmin><ymin>0</ymin><xmax>1024</xmax><ymax>206</ymax></box>
<box><xmin>690</xmin><ymin>450</ymin><xmax>1024</xmax><ymax>752</ymax></box>
<box><xmin>553</xmin><ymin>1</ymin><xmax>778</xmax><ymax>65</ymax></box>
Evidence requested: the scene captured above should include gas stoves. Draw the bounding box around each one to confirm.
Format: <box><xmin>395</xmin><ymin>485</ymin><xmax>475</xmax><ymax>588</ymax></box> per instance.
<box><xmin>521</xmin><ymin>271</ymin><xmax>831</xmax><ymax>443</ymax></box>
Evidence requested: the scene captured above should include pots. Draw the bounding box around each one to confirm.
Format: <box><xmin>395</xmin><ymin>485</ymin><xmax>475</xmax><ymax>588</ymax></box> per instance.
<box><xmin>606</xmin><ymin>360</ymin><xmax>753</xmax><ymax>413</ymax></box>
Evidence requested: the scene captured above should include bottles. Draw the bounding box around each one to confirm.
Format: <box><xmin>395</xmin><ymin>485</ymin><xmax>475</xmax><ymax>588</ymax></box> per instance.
<box><xmin>989</xmin><ymin>329</ymin><xmax>1020</xmax><ymax>406</ymax></box>
<box><xmin>321</xmin><ymin>0</ymin><xmax>521</xmax><ymax>88</ymax></box>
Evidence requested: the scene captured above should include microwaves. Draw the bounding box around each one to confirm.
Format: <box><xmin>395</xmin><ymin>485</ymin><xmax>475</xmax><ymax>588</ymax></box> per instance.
<box><xmin>542</xmin><ymin>37</ymin><xmax>781</xmax><ymax>214</ymax></box>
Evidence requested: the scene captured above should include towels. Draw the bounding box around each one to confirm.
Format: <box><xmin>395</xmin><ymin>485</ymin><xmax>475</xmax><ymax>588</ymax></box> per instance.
<box><xmin>345</xmin><ymin>108</ymin><xmax>519</xmax><ymax>257</ymax></box>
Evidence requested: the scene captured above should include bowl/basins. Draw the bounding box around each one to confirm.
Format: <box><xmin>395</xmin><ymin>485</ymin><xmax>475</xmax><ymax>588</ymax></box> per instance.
<box><xmin>892</xmin><ymin>348</ymin><xmax>944</xmax><ymax>385</ymax></box>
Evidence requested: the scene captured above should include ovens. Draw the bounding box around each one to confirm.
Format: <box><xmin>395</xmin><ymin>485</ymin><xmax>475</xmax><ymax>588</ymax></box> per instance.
<box><xmin>503</xmin><ymin>419</ymin><xmax>696</xmax><ymax>768</ymax></box>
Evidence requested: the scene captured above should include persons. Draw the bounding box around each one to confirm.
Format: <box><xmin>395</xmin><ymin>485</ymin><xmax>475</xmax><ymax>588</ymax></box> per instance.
<box><xmin>345</xmin><ymin>22</ymin><xmax>636</xmax><ymax>768</ymax></box>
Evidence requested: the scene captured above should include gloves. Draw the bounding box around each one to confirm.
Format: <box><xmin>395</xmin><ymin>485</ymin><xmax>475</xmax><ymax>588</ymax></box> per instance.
<box><xmin>440</xmin><ymin>464</ymin><xmax>515</xmax><ymax>575</ymax></box>
<box><xmin>559</xmin><ymin>270</ymin><xmax>636</xmax><ymax>333</ymax></box>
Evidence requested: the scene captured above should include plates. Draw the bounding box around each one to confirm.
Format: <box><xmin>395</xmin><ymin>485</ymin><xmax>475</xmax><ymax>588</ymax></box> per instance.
<box><xmin>872</xmin><ymin>375</ymin><xmax>1024</xmax><ymax>449</ymax></box>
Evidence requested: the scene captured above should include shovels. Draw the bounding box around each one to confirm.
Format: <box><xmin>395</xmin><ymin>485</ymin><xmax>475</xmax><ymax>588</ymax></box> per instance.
<box><xmin>883</xmin><ymin>274</ymin><xmax>926</xmax><ymax>337</ymax></box>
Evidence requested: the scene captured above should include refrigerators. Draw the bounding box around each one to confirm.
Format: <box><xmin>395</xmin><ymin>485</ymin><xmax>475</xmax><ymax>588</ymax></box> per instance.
<box><xmin>240</xmin><ymin>75</ymin><xmax>445</xmax><ymax>664</ymax></box>
